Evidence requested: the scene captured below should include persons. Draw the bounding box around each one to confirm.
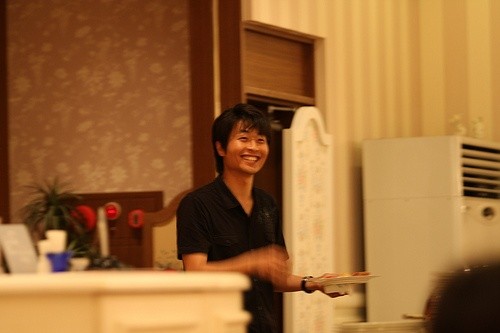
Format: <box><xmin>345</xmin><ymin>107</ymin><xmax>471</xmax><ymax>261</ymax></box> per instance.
<box><xmin>423</xmin><ymin>260</ymin><xmax>500</xmax><ymax>333</ymax></box>
<box><xmin>177</xmin><ymin>103</ymin><xmax>371</xmax><ymax>333</ymax></box>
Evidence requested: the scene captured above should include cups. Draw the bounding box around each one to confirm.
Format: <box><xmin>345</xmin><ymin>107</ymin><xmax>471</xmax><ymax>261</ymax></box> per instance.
<box><xmin>46</xmin><ymin>253</ymin><xmax>73</xmax><ymax>271</ymax></box>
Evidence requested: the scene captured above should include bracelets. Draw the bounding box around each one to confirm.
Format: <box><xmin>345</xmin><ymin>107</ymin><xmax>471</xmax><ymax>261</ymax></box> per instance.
<box><xmin>301</xmin><ymin>276</ymin><xmax>316</xmax><ymax>294</ymax></box>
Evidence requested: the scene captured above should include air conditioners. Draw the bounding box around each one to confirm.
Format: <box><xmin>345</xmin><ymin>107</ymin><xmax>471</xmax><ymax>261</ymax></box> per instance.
<box><xmin>362</xmin><ymin>136</ymin><xmax>500</xmax><ymax>322</ymax></box>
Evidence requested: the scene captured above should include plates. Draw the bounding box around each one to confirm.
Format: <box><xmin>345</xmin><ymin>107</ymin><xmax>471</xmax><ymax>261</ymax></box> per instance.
<box><xmin>303</xmin><ymin>275</ymin><xmax>384</xmax><ymax>286</ymax></box>
